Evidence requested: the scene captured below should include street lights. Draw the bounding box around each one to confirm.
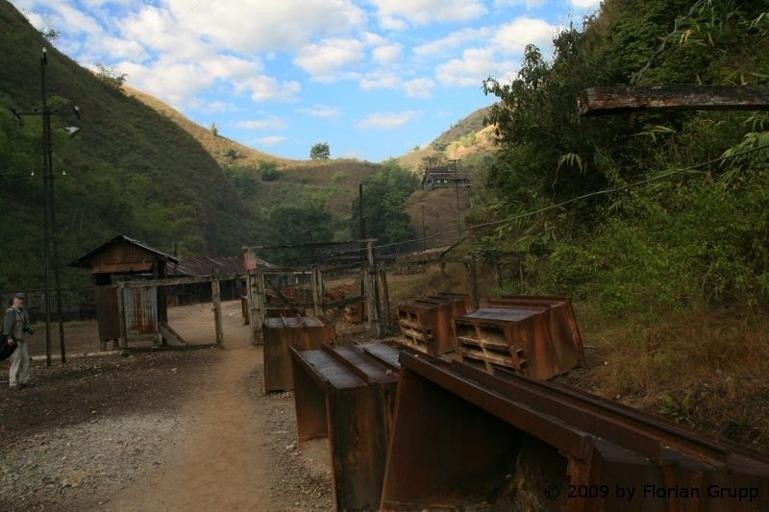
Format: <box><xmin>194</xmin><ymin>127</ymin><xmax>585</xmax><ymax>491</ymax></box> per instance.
<box><xmin>8</xmin><ymin>46</ymin><xmax>81</xmax><ymax>366</ymax></box>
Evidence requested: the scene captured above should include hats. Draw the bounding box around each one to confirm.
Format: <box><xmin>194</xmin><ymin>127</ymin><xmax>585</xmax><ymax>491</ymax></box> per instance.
<box><xmin>15</xmin><ymin>292</ymin><xmax>27</xmax><ymax>299</ymax></box>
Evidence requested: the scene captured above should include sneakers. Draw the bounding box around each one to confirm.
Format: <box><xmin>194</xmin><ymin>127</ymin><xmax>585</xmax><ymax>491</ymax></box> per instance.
<box><xmin>8</xmin><ymin>381</ymin><xmax>36</xmax><ymax>393</ymax></box>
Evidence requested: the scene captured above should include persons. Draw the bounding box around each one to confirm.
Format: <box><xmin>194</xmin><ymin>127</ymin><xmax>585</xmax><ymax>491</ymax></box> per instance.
<box><xmin>3</xmin><ymin>292</ymin><xmax>36</xmax><ymax>389</ymax></box>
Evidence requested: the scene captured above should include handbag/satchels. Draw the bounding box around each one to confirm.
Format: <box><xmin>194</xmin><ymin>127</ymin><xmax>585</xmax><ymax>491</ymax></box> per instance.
<box><xmin>0</xmin><ymin>330</ymin><xmax>18</xmax><ymax>361</ymax></box>
